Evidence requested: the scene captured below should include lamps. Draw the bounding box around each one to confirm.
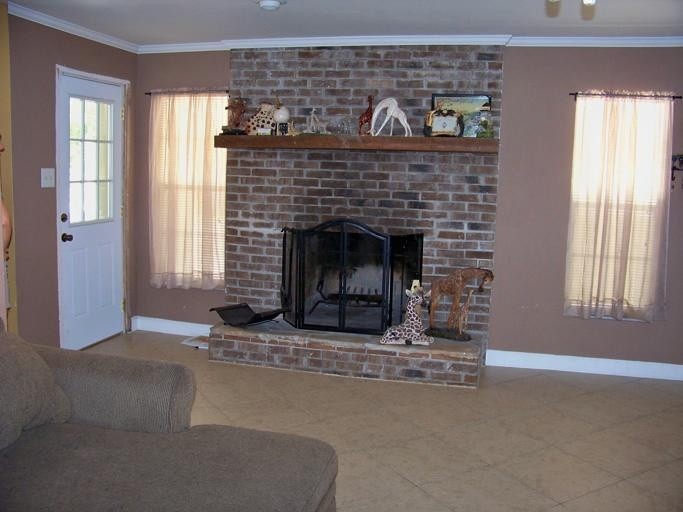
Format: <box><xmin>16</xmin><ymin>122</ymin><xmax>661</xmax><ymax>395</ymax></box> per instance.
<box><xmin>583</xmin><ymin>0</ymin><xmax>596</xmax><ymax>7</ymax></box>
<box><xmin>256</xmin><ymin>0</ymin><xmax>288</xmax><ymax>11</ymax></box>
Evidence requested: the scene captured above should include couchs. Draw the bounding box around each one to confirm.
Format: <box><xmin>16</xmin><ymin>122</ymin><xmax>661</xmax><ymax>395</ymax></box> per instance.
<box><xmin>0</xmin><ymin>316</ymin><xmax>338</xmax><ymax>512</ymax></box>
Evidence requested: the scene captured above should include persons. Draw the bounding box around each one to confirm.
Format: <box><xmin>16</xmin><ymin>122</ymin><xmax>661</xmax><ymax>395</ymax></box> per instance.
<box><xmin>0</xmin><ymin>199</ymin><xmax>12</xmax><ymax>331</ymax></box>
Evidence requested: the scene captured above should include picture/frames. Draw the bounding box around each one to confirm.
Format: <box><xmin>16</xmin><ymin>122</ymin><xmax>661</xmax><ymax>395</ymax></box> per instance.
<box><xmin>431</xmin><ymin>93</ymin><xmax>490</xmax><ymax>138</ymax></box>
<box><xmin>425</xmin><ymin>109</ymin><xmax>465</xmax><ymax>136</ymax></box>
